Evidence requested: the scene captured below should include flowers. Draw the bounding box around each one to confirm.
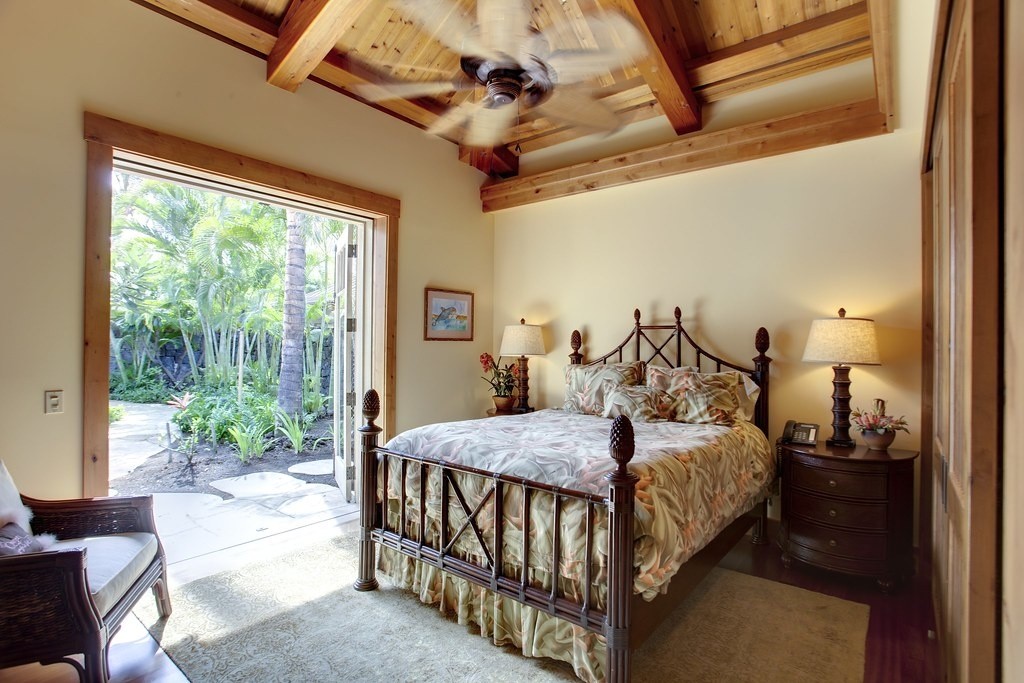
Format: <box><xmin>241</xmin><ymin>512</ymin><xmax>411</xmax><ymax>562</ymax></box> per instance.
<box><xmin>851</xmin><ymin>399</ymin><xmax>911</xmax><ymax>436</ymax></box>
<box><xmin>480</xmin><ymin>353</ymin><xmax>520</xmax><ymax>398</ymax></box>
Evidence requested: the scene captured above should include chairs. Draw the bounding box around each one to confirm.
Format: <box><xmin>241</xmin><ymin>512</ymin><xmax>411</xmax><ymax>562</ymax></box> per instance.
<box><xmin>0</xmin><ymin>459</ymin><xmax>173</xmax><ymax>683</ymax></box>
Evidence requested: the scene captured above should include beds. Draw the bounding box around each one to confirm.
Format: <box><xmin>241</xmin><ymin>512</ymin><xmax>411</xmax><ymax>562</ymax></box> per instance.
<box><xmin>353</xmin><ymin>306</ymin><xmax>768</xmax><ymax>683</ymax></box>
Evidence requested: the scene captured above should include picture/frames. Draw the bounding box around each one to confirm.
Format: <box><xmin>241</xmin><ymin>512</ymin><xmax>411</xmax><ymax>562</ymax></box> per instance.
<box><xmin>424</xmin><ymin>287</ymin><xmax>475</xmax><ymax>341</ymax></box>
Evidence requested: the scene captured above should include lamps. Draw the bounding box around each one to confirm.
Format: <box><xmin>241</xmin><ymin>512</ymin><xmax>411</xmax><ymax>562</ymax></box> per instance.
<box><xmin>498</xmin><ymin>318</ymin><xmax>546</xmax><ymax>415</ymax></box>
<box><xmin>801</xmin><ymin>308</ymin><xmax>882</xmax><ymax>448</ymax></box>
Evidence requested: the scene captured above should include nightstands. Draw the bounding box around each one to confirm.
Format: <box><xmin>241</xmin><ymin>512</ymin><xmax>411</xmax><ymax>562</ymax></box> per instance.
<box><xmin>781</xmin><ymin>443</ymin><xmax>920</xmax><ymax>598</ymax></box>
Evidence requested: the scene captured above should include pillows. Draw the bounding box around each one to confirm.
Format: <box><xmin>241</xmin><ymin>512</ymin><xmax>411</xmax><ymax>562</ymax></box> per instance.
<box><xmin>563</xmin><ymin>361</ymin><xmax>646</xmax><ymax>419</ymax></box>
<box><xmin>676</xmin><ymin>370</ymin><xmax>739</xmax><ymax>425</ymax></box>
<box><xmin>602</xmin><ymin>376</ymin><xmax>678</xmax><ymax>421</ymax></box>
<box><xmin>647</xmin><ymin>364</ymin><xmax>700</xmax><ymax>389</ymax></box>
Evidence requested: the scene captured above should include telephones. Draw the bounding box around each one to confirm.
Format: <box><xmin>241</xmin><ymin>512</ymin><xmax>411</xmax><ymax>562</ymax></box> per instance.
<box><xmin>782</xmin><ymin>420</ymin><xmax>820</xmax><ymax>445</ymax></box>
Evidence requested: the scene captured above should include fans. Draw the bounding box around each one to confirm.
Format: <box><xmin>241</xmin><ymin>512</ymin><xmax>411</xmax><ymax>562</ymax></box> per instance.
<box><xmin>355</xmin><ymin>0</ymin><xmax>651</xmax><ymax>151</ymax></box>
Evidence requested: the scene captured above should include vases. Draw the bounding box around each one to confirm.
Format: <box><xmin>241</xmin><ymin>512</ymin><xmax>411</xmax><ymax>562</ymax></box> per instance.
<box><xmin>492</xmin><ymin>395</ymin><xmax>516</xmax><ymax>411</ymax></box>
<box><xmin>861</xmin><ymin>429</ymin><xmax>896</xmax><ymax>451</ymax></box>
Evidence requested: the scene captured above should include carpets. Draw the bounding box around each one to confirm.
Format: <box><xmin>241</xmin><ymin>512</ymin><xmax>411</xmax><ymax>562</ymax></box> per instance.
<box><xmin>131</xmin><ymin>531</ymin><xmax>871</xmax><ymax>683</ymax></box>
